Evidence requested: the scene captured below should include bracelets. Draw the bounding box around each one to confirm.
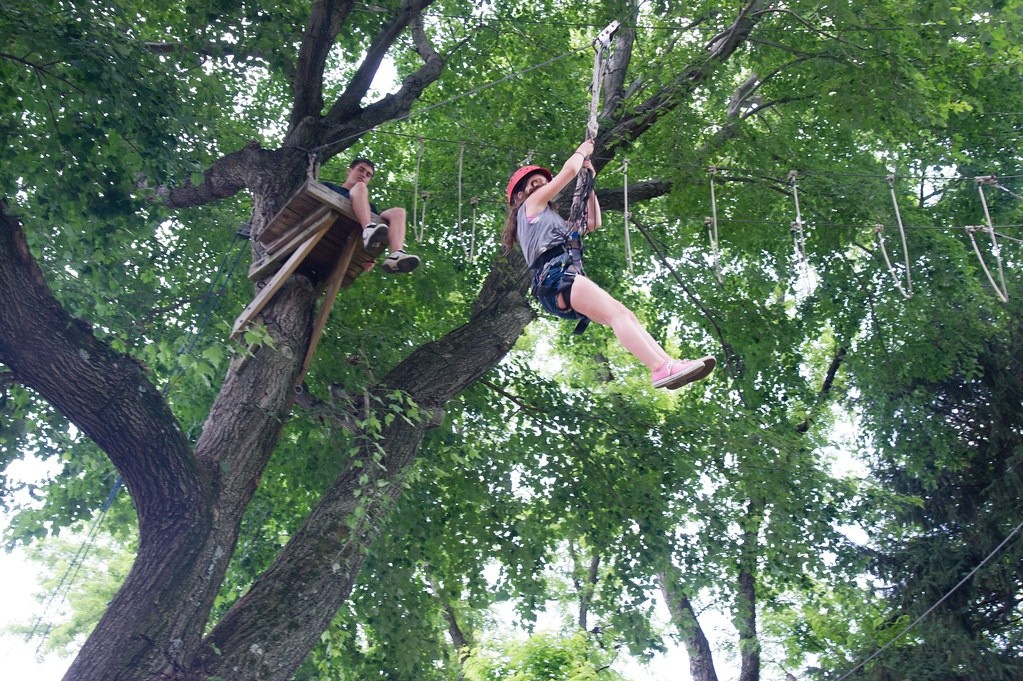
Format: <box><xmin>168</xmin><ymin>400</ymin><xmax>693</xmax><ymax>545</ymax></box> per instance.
<box><xmin>575</xmin><ymin>151</ymin><xmax>585</xmax><ymax>158</ymax></box>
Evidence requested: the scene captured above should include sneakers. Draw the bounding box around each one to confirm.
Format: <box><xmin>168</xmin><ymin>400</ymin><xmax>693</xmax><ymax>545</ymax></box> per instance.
<box><xmin>360</xmin><ymin>222</ymin><xmax>389</xmax><ymax>250</ymax></box>
<box><xmin>381</xmin><ymin>249</ymin><xmax>420</xmax><ymax>274</ymax></box>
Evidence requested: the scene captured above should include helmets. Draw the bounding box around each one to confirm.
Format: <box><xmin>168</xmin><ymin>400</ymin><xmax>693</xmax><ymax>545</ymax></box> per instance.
<box><xmin>507</xmin><ymin>164</ymin><xmax>552</xmax><ymax>206</ymax></box>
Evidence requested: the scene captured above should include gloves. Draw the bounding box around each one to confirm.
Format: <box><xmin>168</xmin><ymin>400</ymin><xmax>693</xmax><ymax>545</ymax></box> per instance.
<box><xmin>575</xmin><ymin>139</ymin><xmax>594</xmax><ymax>157</ymax></box>
<box><xmin>584</xmin><ymin>159</ymin><xmax>596</xmax><ymax>179</ymax></box>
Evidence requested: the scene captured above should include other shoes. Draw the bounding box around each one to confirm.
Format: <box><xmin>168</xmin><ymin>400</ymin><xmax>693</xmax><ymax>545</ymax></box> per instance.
<box><xmin>652</xmin><ymin>360</ymin><xmax>705</xmax><ymax>389</ymax></box>
<box><xmin>667</xmin><ymin>356</ymin><xmax>717</xmax><ymax>390</ymax></box>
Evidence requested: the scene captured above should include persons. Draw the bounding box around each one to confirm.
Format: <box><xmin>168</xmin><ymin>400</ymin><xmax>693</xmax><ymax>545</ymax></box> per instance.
<box><xmin>321</xmin><ymin>159</ymin><xmax>421</xmax><ymax>273</ymax></box>
<box><xmin>503</xmin><ymin>139</ymin><xmax>716</xmax><ymax>390</ymax></box>
